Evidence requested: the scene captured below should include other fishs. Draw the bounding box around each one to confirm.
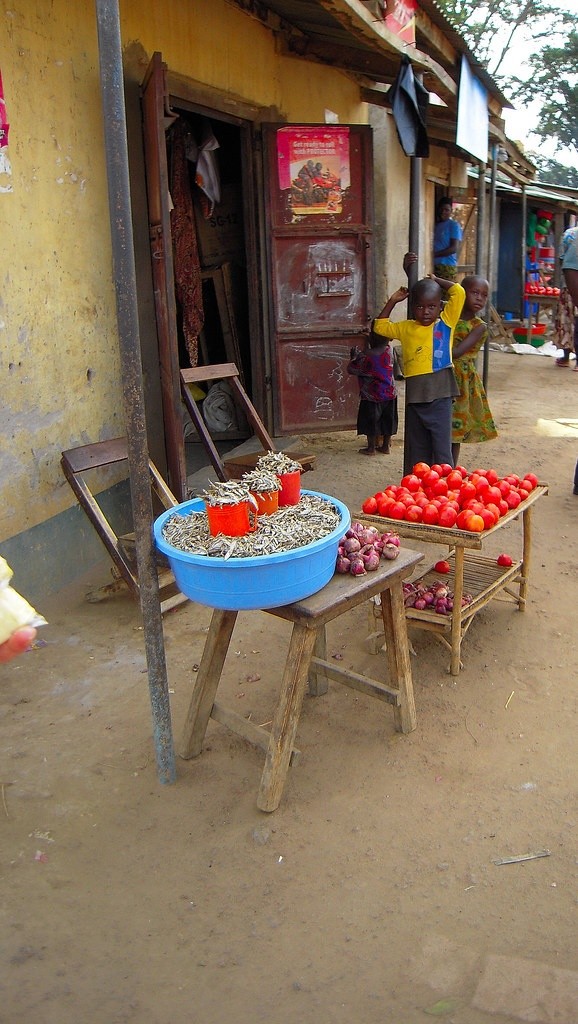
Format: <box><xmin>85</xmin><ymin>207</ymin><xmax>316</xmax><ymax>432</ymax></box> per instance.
<box><xmin>161</xmin><ymin>449</ymin><xmax>342</xmax><ymax>562</ymax></box>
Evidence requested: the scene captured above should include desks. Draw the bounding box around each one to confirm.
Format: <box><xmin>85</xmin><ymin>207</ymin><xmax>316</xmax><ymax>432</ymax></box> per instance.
<box><xmin>525</xmin><ymin>294</ymin><xmax>553</xmax><ymax>346</ymax></box>
<box><xmin>178</xmin><ymin>545</ymin><xmax>425</xmax><ymax>814</ymax></box>
<box><xmin>351</xmin><ymin>481</ymin><xmax>551</xmax><ymax>675</ymax></box>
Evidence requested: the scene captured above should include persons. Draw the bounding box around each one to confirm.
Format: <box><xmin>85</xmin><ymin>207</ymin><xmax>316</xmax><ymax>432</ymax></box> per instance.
<box><xmin>371</xmin><ymin>273</ymin><xmax>466</xmax><ymax>476</ymax></box>
<box><xmin>435</xmin><ymin>197</ymin><xmax>463</xmax><ymax>309</ymax></box>
<box><xmin>552</xmin><ymin>226</ymin><xmax>578</xmax><ymax>371</ymax></box>
<box><xmin>298</xmin><ymin>160</ymin><xmax>322</xmax><ymax>196</ymax></box>
<box><xmin>347</xmin><ymin>319</ymin><xmax>398</xmax><ymax>455</ymax></box>
<box><xmin>403</xmin><ymin>252</ymin><xmax>498</xmax><ymax>468</ymax></box>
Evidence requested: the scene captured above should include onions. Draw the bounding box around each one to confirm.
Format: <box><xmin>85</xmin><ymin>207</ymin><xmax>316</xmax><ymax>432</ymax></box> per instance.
<box><xmin>401</xmin><ymin>581</ymin><xmax>473</xmax><ymax>616</ymax></box>
<box><xmin>335</xmin><ymin>522</ymin><xmax>401</xmax><ymax>577</ymax></box>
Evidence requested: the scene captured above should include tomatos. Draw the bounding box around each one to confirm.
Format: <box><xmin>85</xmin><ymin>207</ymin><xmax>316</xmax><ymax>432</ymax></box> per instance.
<box><xmin>496</xmin><ymin>553</ymin><xmax>512</xmax><ymax>566</ymax></box>
<box><xmin>434</xmin><ymin>560</ymin><xmax>450</xmax><ymax>574</ymax></box>
<box><xmin>360</xmin><ymin>461</ymin><xmax>538</xmax><ymax>533</ymax></box>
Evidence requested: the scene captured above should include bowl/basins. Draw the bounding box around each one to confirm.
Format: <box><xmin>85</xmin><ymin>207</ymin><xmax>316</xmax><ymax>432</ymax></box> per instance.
<box><xmin>513</xmin><ymin>324</ymin><xmax>546</xmax><ymax>335</ymax></box>
<box><xmin>154</xmin><ymin>489</ymin><xmax>352</xmax><ymax>612</ymax></box>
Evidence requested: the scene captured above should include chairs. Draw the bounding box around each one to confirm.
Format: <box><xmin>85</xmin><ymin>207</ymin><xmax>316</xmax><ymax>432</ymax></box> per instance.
<box><xmin>528</xmin><ymin>268</ymin><xmax>552</xmax><ymax>323</ymax></box>
<box><xmin>179</xmin><ymin>363</ymin><xmax>317</xmax><ymax>483</ymax></box>
<box><xmin>60</xmin><ymin>436</ymin><xmax>188</xmax><ymax>620</ymax></box>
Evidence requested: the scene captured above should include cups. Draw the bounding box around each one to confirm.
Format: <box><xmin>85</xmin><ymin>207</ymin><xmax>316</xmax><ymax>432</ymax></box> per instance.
<box><xmin>206</xmin><ymin>499</ymin><xmax>257</xmax><ymax>537</ymax></box>
<box><xmin>276</xmin><ymin>470</ymin><xmax>301</xmax><ymax>506</ymax></box>
<box><xmin>249</xmin><ymin>489</ymin><xmax>279</xmax><ymax>515</ymax></box>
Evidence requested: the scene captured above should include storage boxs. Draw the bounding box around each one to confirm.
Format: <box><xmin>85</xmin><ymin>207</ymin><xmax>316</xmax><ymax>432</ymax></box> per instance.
<box><xmin>193</xmin><ymin>182</ymin><xmax>244</xmax><ymax>267</ymax></box>
